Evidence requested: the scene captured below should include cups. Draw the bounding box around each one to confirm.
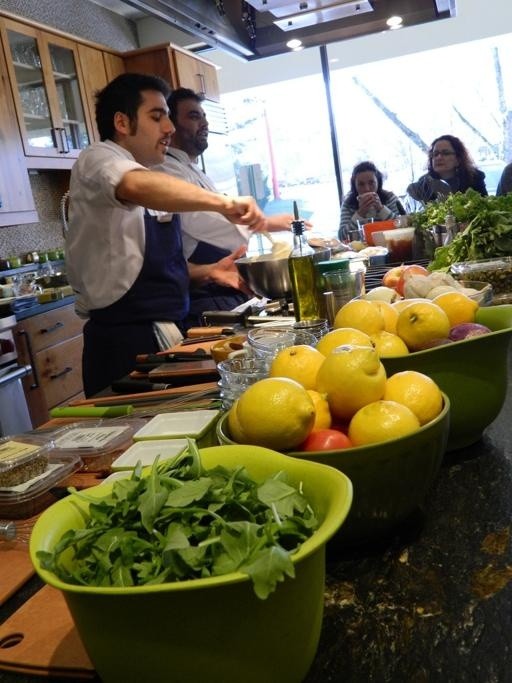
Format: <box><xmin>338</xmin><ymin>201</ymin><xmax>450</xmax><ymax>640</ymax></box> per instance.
<box><xmin>20</xmin><ymin>84</ymin><xmax>67</xmax><ymax>116</ymax></box>
<box><xmin>0</xmin><ymin>246</ymin><xmax>64</xmax><ymax>269</ymax></box>
<box><xmin>10</xmin><ymin>48</ymin><xmax>61</xmax><ymax>72</ymax></box>
<box><xmin>364</xmin><ymin>205</ymin><xmax>376</xmax><ymax>219</ymax></box>
<box><xmin>323</xmin><ymin>269</ymin><xmax>364</xmax><ymax>320</ymax></box>
<box><xmin>384</xmin><ymin>227</ymin><xmax>417</xmax><ymax>264</ymax></box>
<box><xmin>348</xmin><ymin>217</ymin><xmax>375</xmax><ymax>247</ymax></box>
<box><xmin>368</xmin><ymin>255</ymin><xmax>385</xmax><ymax>266</ymax></box>
<box><xmin>1</xmin><ymin>284</ymin><xmax>14</xmax><ymax>297</ymax></box>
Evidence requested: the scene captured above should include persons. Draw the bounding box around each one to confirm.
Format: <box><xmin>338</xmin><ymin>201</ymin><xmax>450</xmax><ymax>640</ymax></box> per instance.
<box><xmin>335</xmin><ymin>159</ymin><xmax>406</xmax><ymax>241</ymax></box>
<box><xmin>154</xmin><ymin>83</ymin><xmax>310</xmax><ymax>320</ymax></box>
<box><xmin>406</xmin><ymin>132</ymin><xmax>490</xmax><ymax>208</ymax></box>
<box><xmin>494</xmin><ymin>160</ymin><xmax>511</xmax><ymax>197</ymax></box>
<box><xmin>68</xmin><ymin>68</ymin><xmax>267</xmax><ymax>398</ymax></box>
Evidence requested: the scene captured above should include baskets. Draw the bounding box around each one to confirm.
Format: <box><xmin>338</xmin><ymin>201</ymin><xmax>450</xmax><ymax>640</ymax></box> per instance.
<box><xmin>365</xmin><ymin>258</ymin><xmax>429</xmax><ymax>291</ymax></box>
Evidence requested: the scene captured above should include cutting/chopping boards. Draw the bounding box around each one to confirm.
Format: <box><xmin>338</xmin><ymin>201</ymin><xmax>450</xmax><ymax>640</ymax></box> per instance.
<box><xmin>129</xmin><ymin>340</ymin><xmax>221</xmax><ymax>379</ymax></box>
<box><xmin>36</xmin><ymin>380</ymin><xmax>218</xmax><ymax>490</ymax></box>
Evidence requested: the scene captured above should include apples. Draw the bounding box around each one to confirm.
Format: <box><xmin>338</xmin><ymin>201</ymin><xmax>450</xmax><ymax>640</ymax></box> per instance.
<box><xmin>381</xmin><ymin>263</ymin><xmax>428</xmax><ymax>292</ymax></box>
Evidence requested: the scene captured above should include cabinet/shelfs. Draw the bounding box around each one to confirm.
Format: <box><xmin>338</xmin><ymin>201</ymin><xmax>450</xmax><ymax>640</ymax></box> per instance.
<box><xmin>13</xmin><ymin>301</ymin><xmax>83</xmax><ymax>427</ymax></box>
<box><xmin>0</xmin><ymin>36</ymin><xmax>38</xmax><ymax>226</ymax></box>
<box><xmin>76</xmin><ymin>42</ymin><xmax>125</xmax><ymax>143</ymax></box>
<box><xmin>125</xmin><ymin>41</ymin><xmax>225</xmax><ymax>103</ymax></box>
<box><xmin>0</xmin><ymin>14</ymin><xmax>94</xmax><ymax>158</ymax></box>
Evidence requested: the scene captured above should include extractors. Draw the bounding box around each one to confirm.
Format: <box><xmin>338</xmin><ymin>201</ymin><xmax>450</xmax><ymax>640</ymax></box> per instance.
<box><xmin>122</xmin><ymin>0</ymin><xmax>456</xmax><ymax>64</ymax></box>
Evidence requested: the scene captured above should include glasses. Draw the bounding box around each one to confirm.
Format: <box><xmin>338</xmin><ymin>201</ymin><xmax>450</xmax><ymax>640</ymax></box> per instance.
<box><xmin>431</xmin><ymin>150</ymin><xmax>456</xmax><ymax>156</ymax></box>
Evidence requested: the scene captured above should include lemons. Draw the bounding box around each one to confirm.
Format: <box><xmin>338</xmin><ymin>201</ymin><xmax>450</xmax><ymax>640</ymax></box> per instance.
<box><xmin>228</xmin><ymin>293</ymin><xmax>481</xmax><ymax>454</ymax></box>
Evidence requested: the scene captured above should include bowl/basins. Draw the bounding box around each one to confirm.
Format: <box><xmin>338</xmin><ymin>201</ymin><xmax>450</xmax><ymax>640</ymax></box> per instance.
<box><xmin>216</xmin><ymin>390</ymin><xmax>451</xmax><ymax>542</ymax></box>
<box><xmin>26</xmin><ymin>444</ymin><xmax>353</xmax><ymax>682</ymax></box>
<box><xmin>460</xmin><ymin>278</ymin><xmax>493</xmax><ymax>306</ymax></box>
<box><xmin>230</xmin><ymin>249</ymin><xmax>338</xmax><ymax>303</ymax></box>
<box><xmin>216</xmin><ymin>319</ymin><xmax>330</xmax><ymax>411</ymax></box>
<box><xmin>378</xmin><ymin>327</ymin><xmax>512</xmax><ymax>445</ymax></box>
<box><xmin>363</xmin><ymin>220</ymin><xmax>394</xmax><ymax>245</ymax></box>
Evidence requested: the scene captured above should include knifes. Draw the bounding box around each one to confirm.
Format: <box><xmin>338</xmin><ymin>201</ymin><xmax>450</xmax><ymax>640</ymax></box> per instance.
<box><xmin>201</xmin><ymin>308</ymin><xmax>299</xmax><ymax>324</ymax></box>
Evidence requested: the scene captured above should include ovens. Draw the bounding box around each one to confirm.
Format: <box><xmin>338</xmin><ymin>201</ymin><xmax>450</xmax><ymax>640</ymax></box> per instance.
<box><xmin>0</xmin><ymin>299</ymin><xmax>36</xmax><ymax>437</ymax></box>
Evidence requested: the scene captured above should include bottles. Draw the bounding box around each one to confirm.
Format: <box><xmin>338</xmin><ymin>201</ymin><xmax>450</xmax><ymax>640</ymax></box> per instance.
<box><xmin>432</xmin><ymin>224</ymin><xmax>442</xmax><ymax>247</ymax></box>
<box><xmin>285</xmin><ymin>201</ymin><xmax>320</xmax><ymax>327</ymax></box>
<box><xmin>443</xmin><ymin>214</ymin><xmax>460</xmax><ymax>246</ymax></box>
<box><xmin>312</xmin><ymin>258</ymin><xmax>350</xmax><ymax>294</ymax></box>
